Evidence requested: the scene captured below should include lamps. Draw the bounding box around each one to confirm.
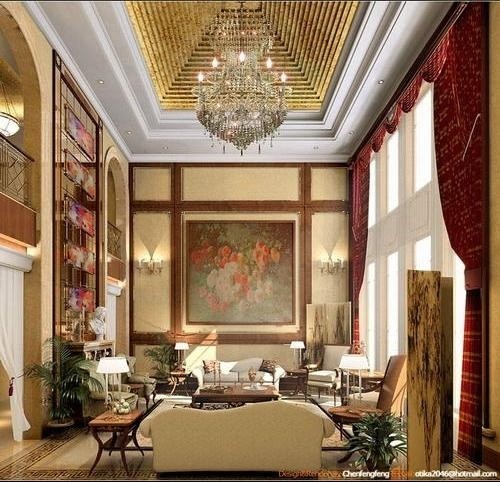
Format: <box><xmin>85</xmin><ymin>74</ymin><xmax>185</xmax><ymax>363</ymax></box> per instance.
<box><xmin>0</xmin><ymin>81</ymin><xmax>20</xmax><ymax>137</ymax></box>
<box><xmin>319</xmin><ymin>257</ymin><xmax>348</xmax><ymax>275</ymax></box>
<box><xmin>191</xmin><ymin>2</ymin><xmax>292</xmax><ymax>156</ymax></box>
<box><xmin>138</xmin><ymin>257</ymin><xmax>164</xmax><ymax>275</ymax></box>
<box><xmin>96</xmin><ymin>356</ymin><xmax>130</xmax><ymax>419</ymax></box>
<box><xmin>338</xmin><ymin>354</ymin><xmax>369</xmax><ymax>399</ymax></box>
<box><xmin>174</xmin><ymin>343</ymin><xmax>189</xmax><ymax>372</ymax></box>
<box><xmin>289</xmin><ymin>340</ymin><xmax>305</xmax><ymax>367</ymax></box>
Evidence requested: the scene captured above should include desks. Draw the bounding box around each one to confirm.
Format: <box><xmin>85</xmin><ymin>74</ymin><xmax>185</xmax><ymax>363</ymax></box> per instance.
<box><xmin>345</xmin><ymin>371</ymin><xmax>384</xmax><ymax>392</ymax></box>
<box><xmin>327</xmin><ymin>406</ymin><xmax>385</xmax><ymax>463</ymax></box>
<box><xmin>129</xmin><ymin>383</ymin><xmax>144</xmax><ymax>408</ymax></box>
<box><xmin>171</xmin><ymin>370</ymin><xmax>191</xmax><ymax>396</ymax></box>
<box><xmin>88</xmin><ymin>409</ymin><xmax>145</xmax><ymax>476</ymax></box>
<box><xmin>286</xmin><ymin>368</ymin><xmax>308</xmax><ymax>402</ymax></box>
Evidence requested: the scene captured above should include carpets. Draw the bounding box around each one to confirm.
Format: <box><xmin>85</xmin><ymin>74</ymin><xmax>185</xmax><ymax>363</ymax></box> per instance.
<box><xmin>101</xmin><ymin>396</ymin><xmax>353</xmax><ymax>451</ymax></box>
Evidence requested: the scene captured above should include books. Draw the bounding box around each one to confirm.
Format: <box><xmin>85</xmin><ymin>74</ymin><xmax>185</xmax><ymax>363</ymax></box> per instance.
<box><xmin>199</xmin><ymin>385</ymin><xmax>233</xmax><ymax>394</ymax></box>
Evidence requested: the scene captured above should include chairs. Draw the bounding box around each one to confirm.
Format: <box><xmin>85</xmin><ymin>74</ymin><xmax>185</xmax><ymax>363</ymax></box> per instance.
<box><xmin>327</xmin><ymin>353</ymin><xmax>408</xmax><ymax>462</ymax></box>
<box><xmin>117</xmin><ymin>352</ymin><xmax>159</xmax><ymax>409</ymax></box>
<box><xmin>79</xmin><ymin>360</ymin><xmax>138</xmax><ymax>433</ymax></box>
<box><xmin>305</xmin><ymin>344</ymin><xmax>350</xmax><ymax>403</ymax></box>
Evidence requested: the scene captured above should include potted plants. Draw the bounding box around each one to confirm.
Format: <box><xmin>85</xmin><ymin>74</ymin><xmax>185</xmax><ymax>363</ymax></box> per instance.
<box><xmin>341</xmin><ymin>409</ymin><xmax>407</xmax><ymax>479</ymax></box>
<box><xmin>143</xmin><ymin>339</ymin><xmax>175</xmax><ymax>394</ymax></box>
<box><xmin>16</xmin><ymin>337</ymin><xmax>104</xmax><ymax>438</ymax></box>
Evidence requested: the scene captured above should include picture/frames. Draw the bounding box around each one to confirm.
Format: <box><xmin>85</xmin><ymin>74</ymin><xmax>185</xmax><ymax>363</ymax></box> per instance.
<box><xmin>186</xmin><ymin>219</ymin><xmax>296</xmax><ymax>325</ymax></box>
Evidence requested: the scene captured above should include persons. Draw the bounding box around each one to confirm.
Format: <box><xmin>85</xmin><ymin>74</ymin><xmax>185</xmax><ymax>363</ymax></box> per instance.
<box><xmin>88</xmin><ymin>307</ymin><xmax>106</xmax><ymax>335</ymax></box>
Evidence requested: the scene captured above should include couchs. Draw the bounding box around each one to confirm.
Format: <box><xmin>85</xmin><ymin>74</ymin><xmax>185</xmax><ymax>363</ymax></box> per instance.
<box><xmin>192</xmin><ymin>357</ymin><xmax>286</xmax><ymax>390</ymax></box>
<box><xmin>139</xmin><ymin>401</ymin><xmax>335</xmax><ymax>477</ymax></box>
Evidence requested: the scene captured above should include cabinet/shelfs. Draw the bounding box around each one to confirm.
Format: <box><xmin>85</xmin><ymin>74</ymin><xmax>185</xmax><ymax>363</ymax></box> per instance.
<box><xmin>63</xmin><ymin>339</ymin><xmax>114</xmax><ymax>427</ymax></box>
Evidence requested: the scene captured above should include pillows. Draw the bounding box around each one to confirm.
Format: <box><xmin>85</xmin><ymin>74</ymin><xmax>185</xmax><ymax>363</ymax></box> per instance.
<box><xmin>259</xmin><ymin>359</ymin><xmax>280</xmax><ymax>372</ymax></box>
<box><xmin>202</xmin><ymin>359</ymin><xmax>221</xmax><ymax>374</ymax></box>
